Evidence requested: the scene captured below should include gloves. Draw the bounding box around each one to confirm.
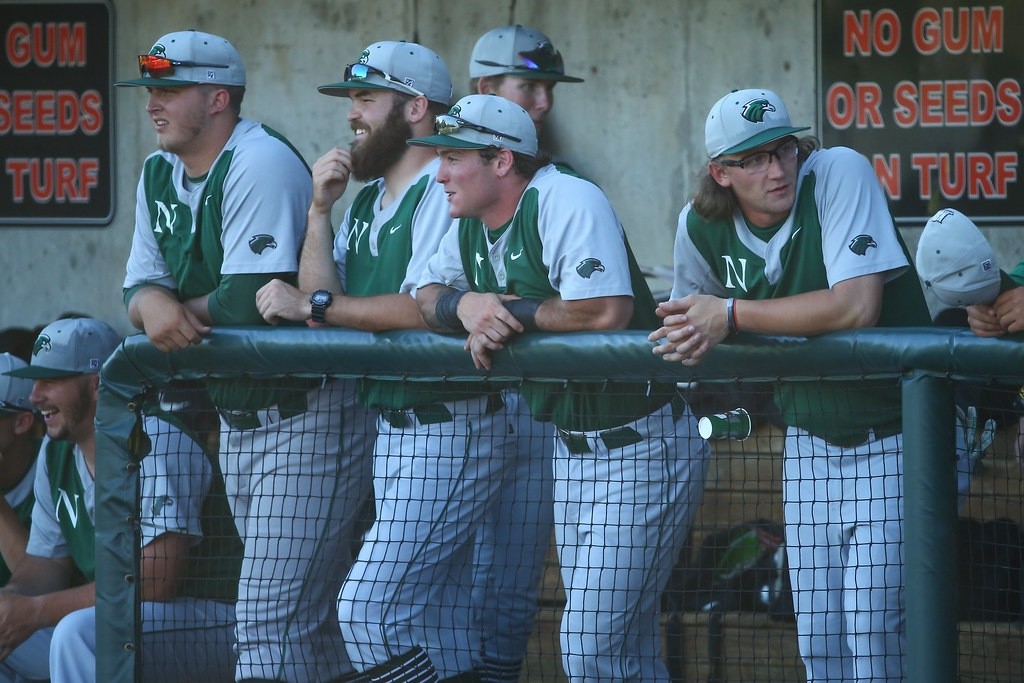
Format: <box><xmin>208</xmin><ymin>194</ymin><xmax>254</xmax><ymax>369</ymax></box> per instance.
<box><xmin>956</xmin><ymin>404</ymin><xmax>997</xmax><ymax>508</ymax></box>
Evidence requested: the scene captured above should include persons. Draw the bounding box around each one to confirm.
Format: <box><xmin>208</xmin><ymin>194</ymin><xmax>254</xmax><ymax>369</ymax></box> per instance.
<box><xmin>652</xmin><ymin>84</ymin><xmax>971</xmax><ymax>682</ymax></box>
<box><xmin>255</xmin><ymin>40</ymin><xmax>520</xmax><ymax>683</ymax></box>
<box><xmin>963</xmin><ymin>256</ymin><xmax>1024</xmax><ymax>342</ymax></box>
<box><xmin>403</xmin><ymin>91</ymin><xmax>708</xmax><ymax>683</ymax></box>
<box><xmin>1</xmin><ymin>315</ymin><xmax>246</xmax><ymax>683</ymax></box>
<box><xmin>470</xmin><ymin>23</ymin><xmax>585</xmax><ymax>683</ymax></box>
<box><xmin>109</xmin><ymin>26</ymin><xmax>377</xmax><ymax>683</ymax></box>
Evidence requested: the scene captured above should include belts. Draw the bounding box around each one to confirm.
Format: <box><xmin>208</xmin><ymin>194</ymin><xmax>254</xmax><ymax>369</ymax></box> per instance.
<box><xmin>557</xmin><ymin>392</ymin><xmax>685</xmax><ymax>454</ymax></box>
<box><xmin>217</xmin><ymin>393</ymin><xmax>308</xmax><ymax>429</ymax></box>
<box><xmin>380</xmin><ymin>389</ymin><xmax>505</xmax><ymax>428</ymax></box>
<box><xmin>816</xmin><ymin>417</ymin><xmax>902</xmax><ymax>448</ymax></box>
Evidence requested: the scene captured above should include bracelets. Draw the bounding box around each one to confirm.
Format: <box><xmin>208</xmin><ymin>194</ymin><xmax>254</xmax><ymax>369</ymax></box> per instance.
<box><xmin>726</xmin><ymin>295</ymin><xmax>739</xmax><ymax>338</ymax></box>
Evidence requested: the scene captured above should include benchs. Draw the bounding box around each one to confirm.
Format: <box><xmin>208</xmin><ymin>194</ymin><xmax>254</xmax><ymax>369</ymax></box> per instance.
<box><xmin>520</xmin><ymin>421</ymin><xmax>1024</xmax><ymax>682</ymax></box>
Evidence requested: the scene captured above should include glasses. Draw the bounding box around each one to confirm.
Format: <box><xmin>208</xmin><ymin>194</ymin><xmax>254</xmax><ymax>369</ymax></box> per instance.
<box><xmin>344</xmin><ymin>63</ymin><xmax>425</xmax><ymax>97</ymax></box>
<box><xmin>720</xmin><ymin>135</ymin><xmax>799</xmax><ymax>174</ymax></box>
<box><xmin>433</xmin><ymin>112</ymin><xmax>521</xmax><ymax>142</ymax></box>
<box><xmin>474</xmin><ymin>48</ymin><xmax>565</xmax><ymax>75</ymax></box>
<box><xmin>138</xmin><ymin>54</ymin><xmax>229</xmax><ymax>78</ymax></box>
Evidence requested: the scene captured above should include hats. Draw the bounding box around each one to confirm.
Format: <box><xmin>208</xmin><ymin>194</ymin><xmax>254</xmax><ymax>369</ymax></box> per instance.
<box><xmin>113</xmin><ymin>29</ymin><xmax>246</xmax><ymax>86</ymax></box>
<box><xmin>469</xmin><ymin>24</ymin><xmax>584</xmax><ymax>82</ymax></box>
<box><xmin>316</xmin><ymin>40</ymin><xmax>453</xmax><ymax>106</ymax></box>
<box><xmin>916</xmin><ymin>208</ymin><xmax>1019</xmax><ymax>325</ymax></box>
<box><xmin>406</xmin><ymin>93</ymin><xmax>538</xmax><ymax>158</ymax></box>
<box><xmin>705</xmin><ymin>88</ymin><xmax>811</xmax><ymax>159</ymax></box>
<box><xmin>0</xmin><ymin>352</ymin><xmax>42</xmax><ymax>417</ymax></box>
<box><xmin>1</xmin><ymin>315</ymin><xmax>122</xmax><ymax>378</ymax></box>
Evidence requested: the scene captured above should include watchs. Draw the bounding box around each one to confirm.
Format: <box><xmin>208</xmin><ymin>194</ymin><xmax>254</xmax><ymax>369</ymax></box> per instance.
<box><xmin>308</xmin><ymin>287</ymin><xmax>334</xmax><ymax>325</ymax></box>
